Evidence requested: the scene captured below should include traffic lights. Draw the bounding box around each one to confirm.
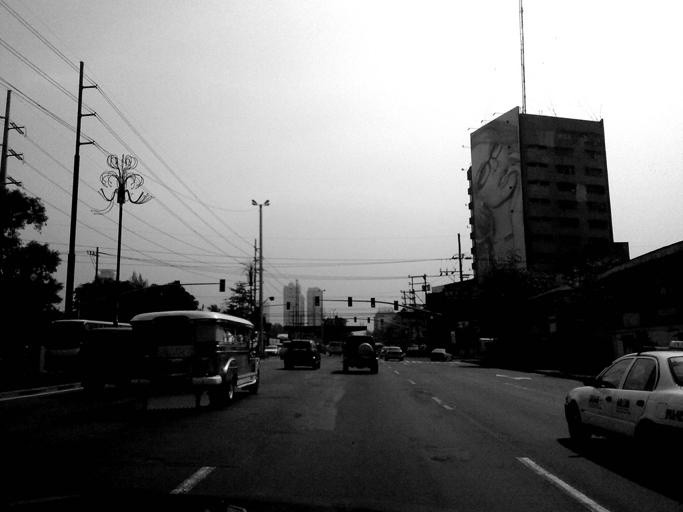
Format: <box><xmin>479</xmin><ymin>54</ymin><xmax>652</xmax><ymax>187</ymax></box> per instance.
<box><xmin>394</xmin><ymin>300</ymin><xmax>398</xmax><ymax>310</ymax></box>
<box><xmin>315</xmin><ymin>296</ymin><xmax>320</xmax><ymax>306</ymax></box>
<box><xmin>371</xmin><ymin>298</ymin><xmax>375</xmax><ymax>308</ymax></box>
<box><xmin>348</xmin><ymin>297</ymin><xmax>352</xmax><ymax>307</ymax></box>
<box><xmin>286</xmin><ymin>301</ymin><xmax>291</xmax><ymax>310</ymax></box>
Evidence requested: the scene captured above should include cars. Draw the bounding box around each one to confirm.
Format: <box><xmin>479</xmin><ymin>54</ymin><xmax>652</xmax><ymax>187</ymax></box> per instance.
<box><xmin>377</xmin><ymin>343</ymin><xmax>403</xmax><ymax>362</ymax></box>
<box><xmin>316</xmin><ymin>342</ymin><xmax>343</xmax><ymax>355</ymax></box>
<box><xmin>264</xmin><ymin>340</ymin><xmax>291</xmax><ymax>359</ymax></box>
<box><xmin>430</xmin><ymin>347</ymin><xmax>453</xmax><ymax>362</ymax></box>
<box><xmin>40</xmin><ymin>310</ymin><xmax>260</xmax><ymax>407</ymax></box>
<box><xmin>564</xmin><ymin>341</ymin><xmax>683</xmax><ymax>460</ymax></box>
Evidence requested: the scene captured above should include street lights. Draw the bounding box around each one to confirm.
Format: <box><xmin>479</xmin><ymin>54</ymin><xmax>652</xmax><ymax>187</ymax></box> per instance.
<box><xmin>219</xmin><ymin>279</ymin><xmax>225</xmax><ymax>292</ymax></box>
<box><xmin>252</xmin><ymin>198</ymin><xmax>270</xmax><ymax>357</ymax></box>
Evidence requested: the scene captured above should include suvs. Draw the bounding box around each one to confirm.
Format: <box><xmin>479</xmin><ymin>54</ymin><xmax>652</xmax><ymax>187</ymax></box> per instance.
<box><xmin>284</xmin><ymin>339</ymin><xmax>321</xmax><ymax>370</ymax></box>
<box><xmin>342</xmin><ymin>334</ymin><xmax>378</xmax><ymax>373</ymax></box>
